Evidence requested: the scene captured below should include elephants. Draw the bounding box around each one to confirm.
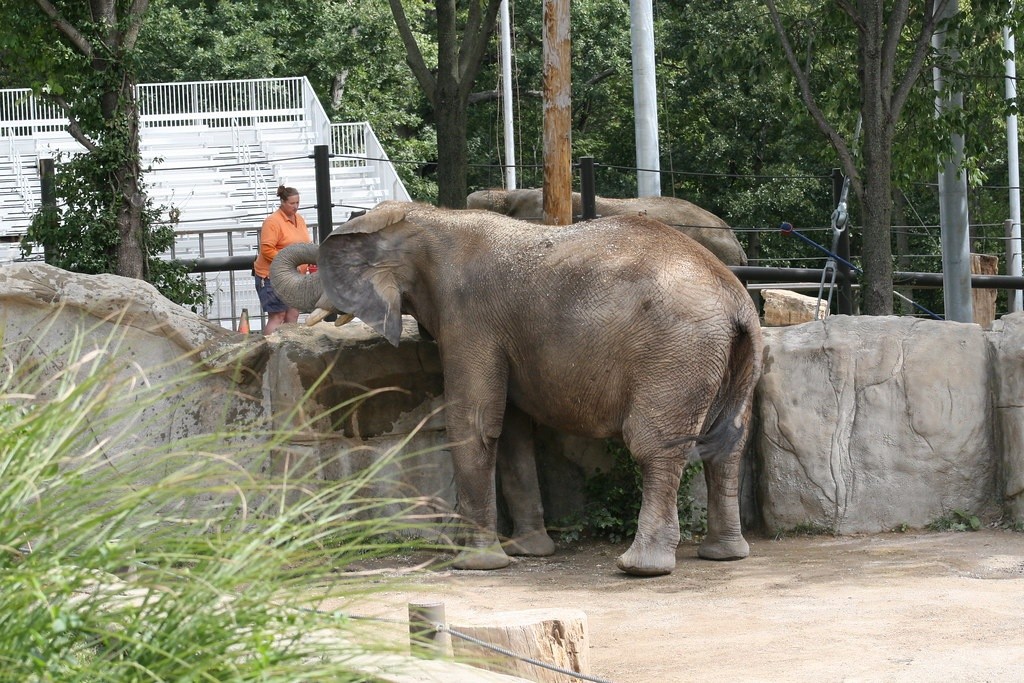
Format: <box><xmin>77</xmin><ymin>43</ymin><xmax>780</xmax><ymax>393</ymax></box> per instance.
<box><xmin>267</xmin><ymin>185</ymin><xmax>764</xmax><ymax>575</ymax></box>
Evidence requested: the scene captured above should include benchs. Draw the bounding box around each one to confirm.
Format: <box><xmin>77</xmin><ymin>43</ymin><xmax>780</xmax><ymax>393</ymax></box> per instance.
<box><xmin>0</xmin><ymin>113</ymin><xmax>390</xmax><ymax>331</ymax></box>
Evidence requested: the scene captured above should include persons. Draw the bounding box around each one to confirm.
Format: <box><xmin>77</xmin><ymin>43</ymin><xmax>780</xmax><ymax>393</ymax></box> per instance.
<box><xmin>253</xmin><ymin>185</ymin><xmax>316</xmax><ymax>335</ymax></box>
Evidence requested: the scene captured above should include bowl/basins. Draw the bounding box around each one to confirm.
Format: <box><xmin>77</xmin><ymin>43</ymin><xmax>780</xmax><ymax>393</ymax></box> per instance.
<box><xmin>309</xmin><ymin>267</ymin><xmax>318</xmax><ymax>274</ymax></box>
